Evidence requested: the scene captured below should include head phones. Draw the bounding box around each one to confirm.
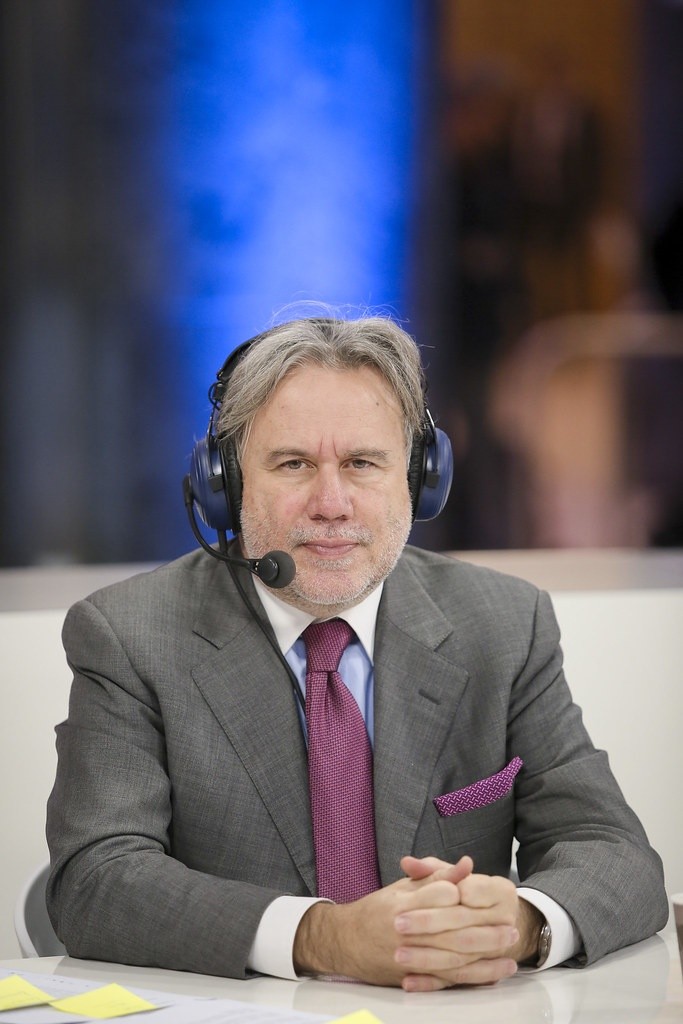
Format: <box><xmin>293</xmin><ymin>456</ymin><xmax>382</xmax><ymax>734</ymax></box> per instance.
<box><xmin>182</xmin><ymin>318</ymin><xmax>455</xmax><ymax>533</ymax></box>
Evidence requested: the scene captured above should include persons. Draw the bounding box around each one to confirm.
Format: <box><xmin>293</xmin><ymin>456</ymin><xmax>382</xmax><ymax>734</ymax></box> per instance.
<box><xmin>44</xmin><ymin>305</ymin><xmax>669</xmax><ymax>991</ymax></box>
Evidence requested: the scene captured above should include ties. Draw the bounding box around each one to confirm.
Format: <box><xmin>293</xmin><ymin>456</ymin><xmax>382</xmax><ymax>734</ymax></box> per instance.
<box><xmin>302</xmin><ymin>620</ymin><xmax>382</xmax><ymax>905</ymax></box>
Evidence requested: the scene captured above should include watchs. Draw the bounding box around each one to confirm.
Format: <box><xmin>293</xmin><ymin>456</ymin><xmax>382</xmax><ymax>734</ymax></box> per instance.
<box><xmin>538</xmin><ymin>915</ymin><xmax>551</xmax><ymax>968</ymax></box>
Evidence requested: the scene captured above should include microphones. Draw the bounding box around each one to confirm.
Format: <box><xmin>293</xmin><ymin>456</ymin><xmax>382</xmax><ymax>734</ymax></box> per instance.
<box><xmin>186</xmin><ymin>503</ymin><xmax>297</xmax><ymax>588</ymax></box>
